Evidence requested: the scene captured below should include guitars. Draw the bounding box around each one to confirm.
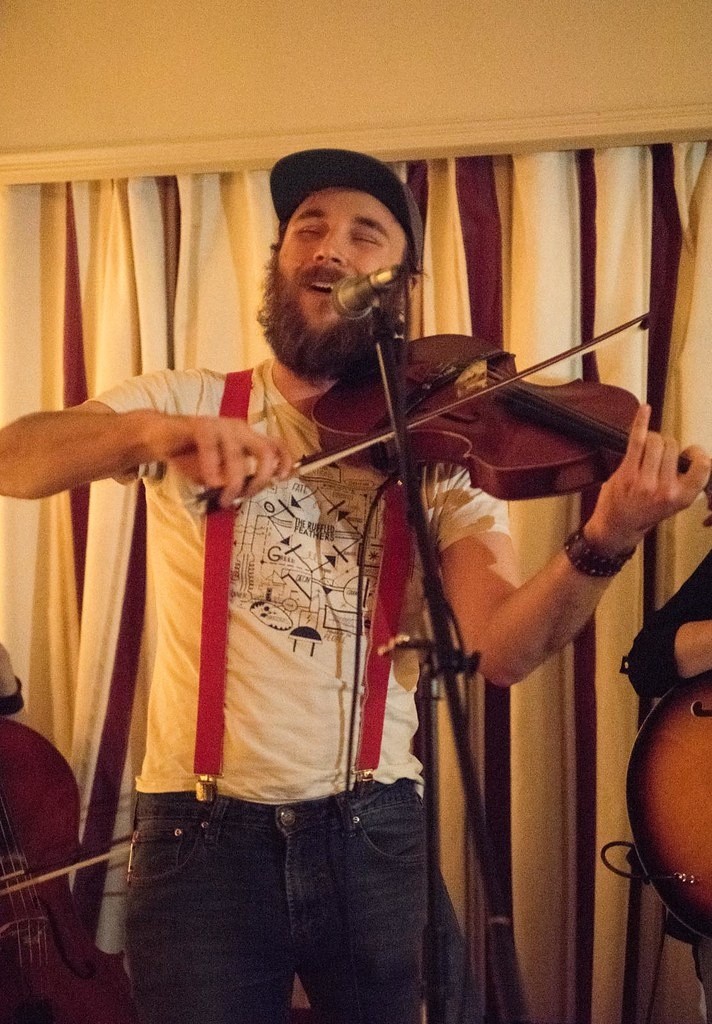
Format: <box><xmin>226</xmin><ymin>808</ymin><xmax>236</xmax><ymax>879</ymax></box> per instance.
<box><xmin>625</xmin><ymin>665</ymin><xmax>712</xmax><ymax>942</ymax></box>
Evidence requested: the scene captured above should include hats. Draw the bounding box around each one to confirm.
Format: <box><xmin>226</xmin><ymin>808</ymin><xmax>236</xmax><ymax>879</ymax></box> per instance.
<box><xmin>270</xmin><ymin>148</ymin><xmax>423</xmax><ymax>272</ymax></box>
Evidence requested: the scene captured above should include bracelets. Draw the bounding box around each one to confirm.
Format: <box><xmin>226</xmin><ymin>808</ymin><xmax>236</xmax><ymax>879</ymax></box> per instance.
<box><xmin>558</xmin><ymin>521</ymin><xmax>635</xmax><ymax>581</ymax></box>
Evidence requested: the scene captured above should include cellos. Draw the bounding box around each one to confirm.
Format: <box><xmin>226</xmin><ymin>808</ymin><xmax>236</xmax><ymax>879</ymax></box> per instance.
<box><xmin>0</xmin><ymin>712</ymin><xmax>134</xmax><ymax>1024</ymax></box>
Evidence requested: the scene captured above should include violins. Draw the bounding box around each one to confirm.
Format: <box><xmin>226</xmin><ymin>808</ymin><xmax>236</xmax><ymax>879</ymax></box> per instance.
<box><xmin>310</xmin><ymin>330</ymin><xmax>712</xmax><ymax>525</ymax></box>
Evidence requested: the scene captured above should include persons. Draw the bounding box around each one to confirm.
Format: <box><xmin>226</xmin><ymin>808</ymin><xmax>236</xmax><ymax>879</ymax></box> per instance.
<box><xmin>0</xmin><ymin>146</ymin><xmax>711</xmax><ymax>1023</ymax></box>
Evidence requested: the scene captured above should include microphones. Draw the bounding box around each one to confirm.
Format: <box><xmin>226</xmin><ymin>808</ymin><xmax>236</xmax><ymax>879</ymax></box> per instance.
<box><xmin>330</xmin><ymin>264</ymin><xmax>401</xmax><ymax>320</ymax></box>
